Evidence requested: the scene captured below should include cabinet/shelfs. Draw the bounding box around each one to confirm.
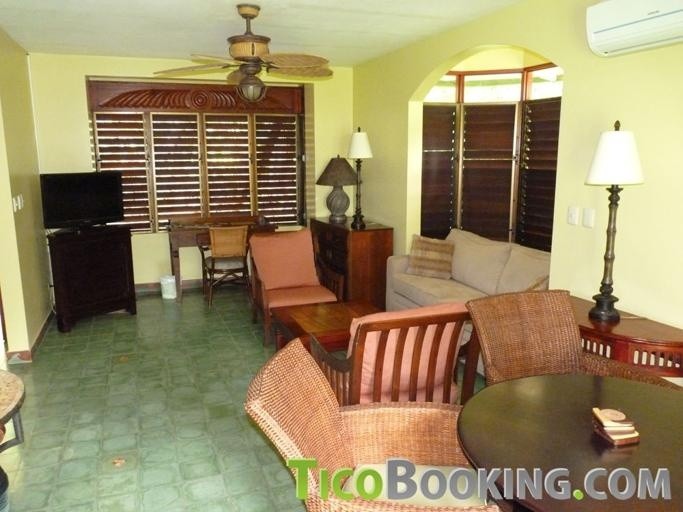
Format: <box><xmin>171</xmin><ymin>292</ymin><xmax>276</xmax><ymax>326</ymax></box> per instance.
<box><xmin>313</xmin><ymin>215</ymin><xmax>393</xmax><ymax>308</ymax></box>
<box><xmin>46</xmin><ymin>222</ymin><xmax>137</xmax><ymax>331</ymax></box>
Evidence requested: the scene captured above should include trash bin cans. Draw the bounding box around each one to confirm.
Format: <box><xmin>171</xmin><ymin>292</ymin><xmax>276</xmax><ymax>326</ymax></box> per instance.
<box><xmin>160</xmin><ymin>275</ymin><xmax>177</xmax><ymax>299</ymax></box>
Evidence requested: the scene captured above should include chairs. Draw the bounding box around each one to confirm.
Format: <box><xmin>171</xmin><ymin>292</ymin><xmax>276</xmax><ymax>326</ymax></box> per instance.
<box><xmin>242</xmin><ymin>333</ymin><xmax>502</xmax><ymax>512</ymax></box>
<box><xmin>245</xmin><ymin>225</ymin><xmax>340</xmax><ymax>346</ymax></box>
<box><xmin>197</xmin><ymin>219</ymin><xmax>251</xmax><ymax>308</ymax></box>
<box><xmin>311</xmin><ymin>302</ymin><xmax>476</xmax><ymax>410</ymax></box>
<box><xmin>464</xmin><ymin>289</ymin><xmax>682</xmax><ymax>391</ymax></box>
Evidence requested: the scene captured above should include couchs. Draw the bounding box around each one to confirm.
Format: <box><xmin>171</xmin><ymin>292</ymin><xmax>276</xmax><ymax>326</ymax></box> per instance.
<box><xmin>379</xmin><ymin>228</ymin><xmax>551</xmax><ymax>324</ymax></box>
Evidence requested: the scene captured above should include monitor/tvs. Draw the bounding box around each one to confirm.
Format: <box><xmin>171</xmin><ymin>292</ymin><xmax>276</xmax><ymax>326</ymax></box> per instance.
<box><xmin>40</xmin><ymin>170</ymin><xmax>124</xmax><ymax>233</ymax></box>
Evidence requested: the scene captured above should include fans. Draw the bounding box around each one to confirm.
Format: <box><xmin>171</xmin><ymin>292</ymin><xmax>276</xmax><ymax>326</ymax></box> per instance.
<box><xmin>145</xmin><ymin>4</ymin><xmax>335</xmax><ymax>88</ymax></box>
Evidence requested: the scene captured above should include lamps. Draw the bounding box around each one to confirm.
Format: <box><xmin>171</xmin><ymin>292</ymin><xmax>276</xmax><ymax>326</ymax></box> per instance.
<box><xmin>236</xmin><ymin>66</ymin><xmax>266</xmax><ymax>105</ymax></box>
<box><xmin>349</xmin><ymin>124</ymin><xmax>374</xmax><ymax>230</ymax></box>
<box><xmin>315</xmin><ymin>154</ymin><xmax>363</xmax><ymax>222</ymax></box>
<box><xmin>578</xmin><ymin>117</ymin><xmax>644</xmax><ymax>326</ymax></box>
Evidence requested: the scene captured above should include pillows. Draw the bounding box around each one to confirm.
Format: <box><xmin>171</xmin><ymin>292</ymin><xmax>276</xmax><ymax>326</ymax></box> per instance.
<box><xmin>525</xmin><ymin>275</ymin><xmax>551</xmax><ymax>291</ymax></box>
<box><xmin>405</xmin><ymin>232</ymin><xmax>453</xmax><ymax>279</ymax></box>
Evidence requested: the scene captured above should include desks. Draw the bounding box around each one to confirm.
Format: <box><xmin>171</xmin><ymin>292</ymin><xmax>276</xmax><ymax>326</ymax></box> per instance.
<box><xmin>0</xmin><ymin>366</ymin><xmax>26</xmax><ymax>492</ymax></box>
<box><xmin>165</xmin><ymin>212</ymin><xmax>279</xmax><ymax>303</ymax></box>
<box><xmin>454</xmin><ymin>368</ymin><xmax>682</xmax><ymax>512</ymax></box>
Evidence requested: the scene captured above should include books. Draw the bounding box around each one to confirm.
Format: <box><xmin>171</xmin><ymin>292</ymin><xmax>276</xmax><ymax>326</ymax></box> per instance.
<box><xmin>592</xmin><ymin>406</ymin><xmax>640</xmax><ymax>447</ymax></box>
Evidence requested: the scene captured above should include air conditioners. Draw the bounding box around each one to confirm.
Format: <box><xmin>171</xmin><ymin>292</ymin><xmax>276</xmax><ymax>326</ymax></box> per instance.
<box><xmin>584</xmin><ymin>1</ymin><xmax>682</xmax><ymax>57</ymax></box>
